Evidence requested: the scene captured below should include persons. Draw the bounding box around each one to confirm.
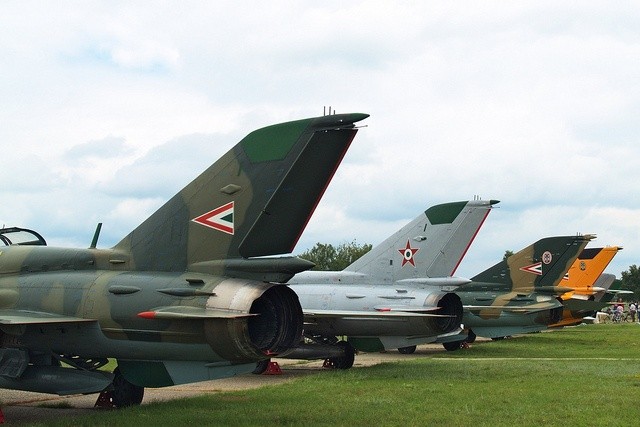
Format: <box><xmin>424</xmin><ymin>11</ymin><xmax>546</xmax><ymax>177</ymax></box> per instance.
<box><xmin>618</xmin><ymin>297</ymin><xmax>625</xmax><ymax>309</ymax></box>
<box><xmin>607</xmin><ymin>307</ymin><xmax>619</xmax><ymax>322</ymax></box>
<box><xmin>629</xmin><ymin>302</ymin><xmax>637</xmax><ymax>322</ymax></box>
<box><xmin>612</xmin><ymin>304</ymin><xmax>618</xmax><ymax>309</ymax></box>
<box><xmin>616</xmin><ymin>305</ymin><xmax>624</xmax><ymax>321</ymax></box>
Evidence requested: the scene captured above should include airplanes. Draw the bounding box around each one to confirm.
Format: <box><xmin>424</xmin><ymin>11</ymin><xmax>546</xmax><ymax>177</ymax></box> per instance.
<box><xmin>0</xmin><ymin>105</ymin><xmax>371</xmax><ymax>410</ymax></box>
<box><xmin>490</xmin><ymin>245</ymin><xmax>623</xmax><ymax>343</ymax></box>
<box><xmin>585</xmin><ymin>273</ymin><xmax>635</xmax><ymax>318</ymax></box>
<box><xmin>252</xmin><ymin>194</ymin><xmax>500</xmax><ymax>375</ymax></box>
<box><xmin>397</xmin><ymin>231</ymin><xmax>596</xmax><ymax>355</ymax></box>
